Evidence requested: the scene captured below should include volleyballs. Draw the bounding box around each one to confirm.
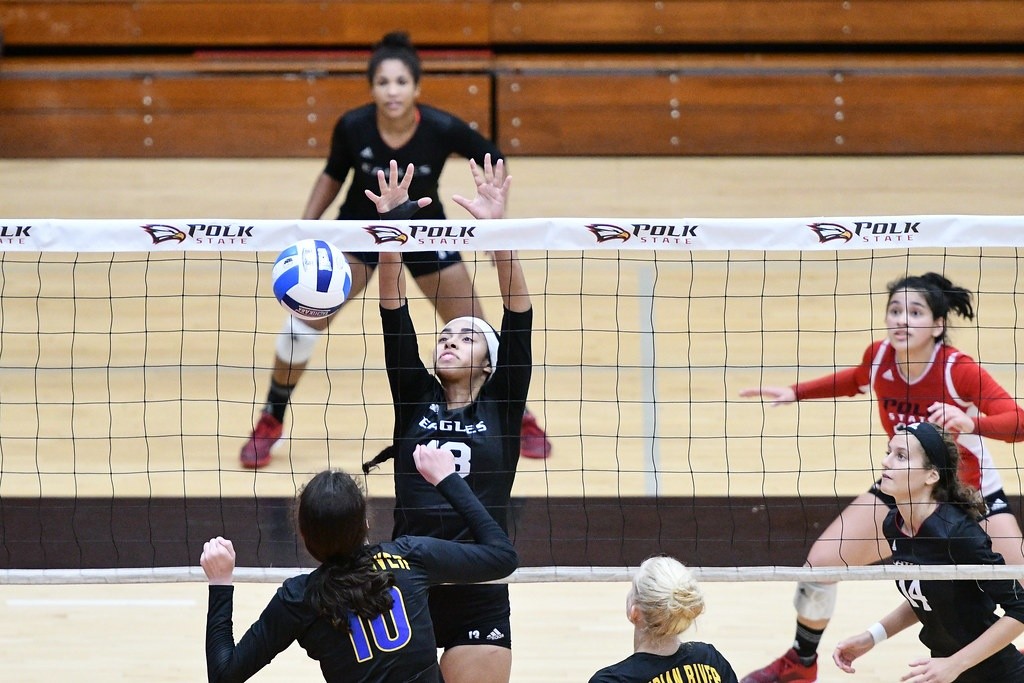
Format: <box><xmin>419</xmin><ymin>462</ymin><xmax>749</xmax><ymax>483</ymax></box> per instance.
<box><xmin>271</xmin><ymin>239</ymin><xmax>353</xmax><ymax>321</ymax></box>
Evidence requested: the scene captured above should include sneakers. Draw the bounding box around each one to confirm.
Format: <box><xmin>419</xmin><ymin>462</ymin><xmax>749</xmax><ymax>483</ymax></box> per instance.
<box><xmin>239</xmin><ymin>409</ymin><xmax>283</xmax><ymax>469</ymax></box>
<box><xmin>740</xmin><ymin>647</ymin><xmax>818</xmax><ymax>683</ymax></box>
<box><xmin>521</xmin><ymin>406</ymin><xmax>553</xmax><ymax>459</ymax></box>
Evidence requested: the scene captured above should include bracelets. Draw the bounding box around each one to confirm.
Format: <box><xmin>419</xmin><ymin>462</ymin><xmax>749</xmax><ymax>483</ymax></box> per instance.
<box><xmin>868</xmin><ymin>622</ymin><xmax>887</xmax><ymax>645</ymax></box>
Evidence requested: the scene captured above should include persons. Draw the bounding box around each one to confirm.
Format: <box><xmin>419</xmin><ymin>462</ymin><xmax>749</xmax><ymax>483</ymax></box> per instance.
<box><xmin>739</xmin><ymin>272</ymin><xmax>1024</xmax><ymax>683</ymax></box>
<box><xmin>363</xmin><ymin>152</ymin><xmax>533</xmax><ymax>683</ymax></box>
<box><xmin>239</xmin><ymin>30</ymin><xmax>552</xmax><ymax>469</ymax></box>
<box><xmin>831</xmin><ymin>422</ymin><xmax>1023</xmax><ymax>683</ymax></box>
<box><xmin>588</xmin><ymin>555</ymin><xmax>739</xmax><ymax>683</ymax></box>
<box><xmin>200</xmin><ymin>440</ymin><xmax>518</xmax><ymax>683</ymax></box>
